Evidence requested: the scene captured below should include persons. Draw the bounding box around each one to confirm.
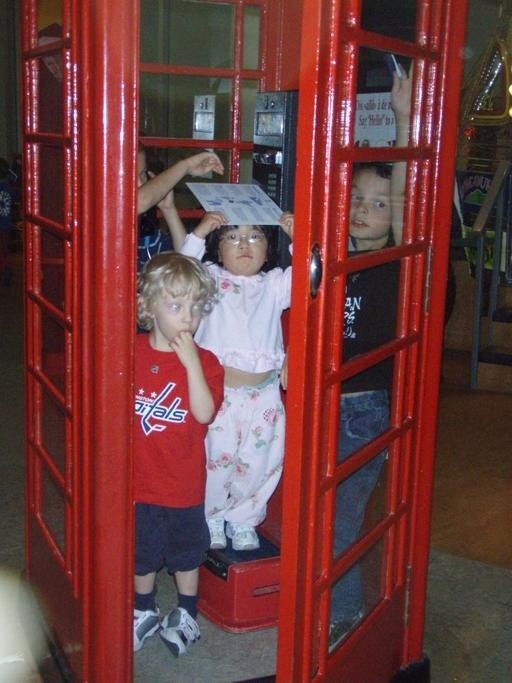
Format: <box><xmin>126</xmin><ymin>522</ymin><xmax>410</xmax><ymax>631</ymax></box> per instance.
<box><xmin>178</xmin><ymin>210</ymin><xmax>293</xmax><ymax>551</ymax></box>
<box><xmin>136</xmin><ymin>251</ymin><xmax>225</xmax><ymax>657</ymax></box>
<box><xmin>138</xmin><ymin>144</ymin><xmax>225</xmax><ymax>270</ymax></box>
<box><xmin>330</xmin><ymin>59</ymin><xmax>413</xmax><ymax>628</ymax></box>
<box><xmin>0</xmin><ymin>159</ymin><xmax>16</xmax><ymax>295</ymax></box>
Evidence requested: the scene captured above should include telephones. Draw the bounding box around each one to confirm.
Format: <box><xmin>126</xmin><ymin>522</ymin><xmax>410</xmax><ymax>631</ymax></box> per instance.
<box><xmin>251</xmin><ymin>90</ymin><xmax>299</xmax><ymax>252</ymax></box>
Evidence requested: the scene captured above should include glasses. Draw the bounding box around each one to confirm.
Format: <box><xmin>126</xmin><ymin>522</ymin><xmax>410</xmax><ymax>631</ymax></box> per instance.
<box><xmin>217</xmin><ymin>230</ymin><xmax>265</xmax><ymax>245</ymax></box>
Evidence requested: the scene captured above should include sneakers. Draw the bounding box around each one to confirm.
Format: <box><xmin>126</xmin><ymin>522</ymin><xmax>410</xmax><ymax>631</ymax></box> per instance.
<box><xmin>328</xmin><ymin>606</ymin><xmax>367</xmax><ymax>654</ymax></box>
<box><xmin>205</xmin><ymin>518</ymin><xmax>227</xmax><ymax>551</ymax></box>
<box><xmin>159</xmin><ymin>607</ymin><xmax>201</xmax><ymax>658</ymax></box>
<box><xmin>225</xmin><ymin>522</ymin><xmax>260</xmax><ymax>551</ymax></box>
<box><xmin>134</xmin><ymin>606</ymin><xmax>161</xmax><ymax>653</ymax></box>
